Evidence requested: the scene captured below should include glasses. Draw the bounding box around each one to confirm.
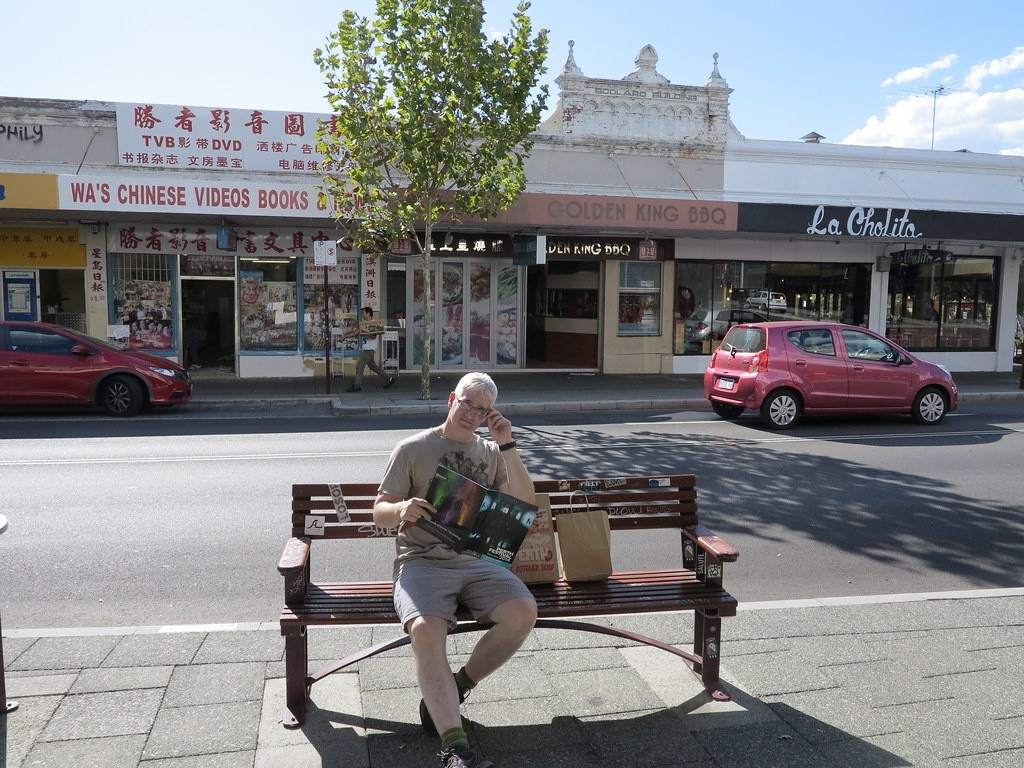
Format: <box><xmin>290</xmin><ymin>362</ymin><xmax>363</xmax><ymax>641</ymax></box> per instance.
<box><xmin>454</xmin><ymin>395</ymin><xmax>491</xmax><ymax>418</ymax></box>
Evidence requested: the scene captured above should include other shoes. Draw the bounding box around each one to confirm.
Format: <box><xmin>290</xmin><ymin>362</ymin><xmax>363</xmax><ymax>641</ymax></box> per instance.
<box><xmin>383</xmin><ymin>377</ymin><xmax>396</xmax><ymax>389</ymax></box>
<box><xmin>347</xmin><ymin>385</ymin><xmax>362</xmax><ymax>393</ymax></box>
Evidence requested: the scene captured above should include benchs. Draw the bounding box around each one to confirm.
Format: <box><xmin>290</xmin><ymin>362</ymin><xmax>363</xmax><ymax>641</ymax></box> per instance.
<box><xmin>277</xmin><ymin>474</ymin><xmax>741</xmax><ymax>728</ymax></box>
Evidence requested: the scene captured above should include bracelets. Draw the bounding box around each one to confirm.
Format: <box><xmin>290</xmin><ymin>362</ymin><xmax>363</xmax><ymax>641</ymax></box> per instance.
<box><xmin>499</xmin><ymin>439</ymin><xmax>518</xmax><ymax>452</ymax></box>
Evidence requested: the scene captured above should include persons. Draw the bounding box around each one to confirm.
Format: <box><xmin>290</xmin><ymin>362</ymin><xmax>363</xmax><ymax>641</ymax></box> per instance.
<box><xmin>372</xmin><ymin>372</ymin><xmax>540</xmax><ymax>768</ymax></box>
<box><xmin>341</xmin><ymin>306</ymin><xmax>395</xmax><ymax>394</ymax></box>
<box><xmin>927</xmin><ymin>297</ymin><xmax>940</xmax><ymax>323</ymax></box>
<box><xmin>120</xmin><ymin>303</ymin><xmax>172</xmax><ymax>347</ymax></box>
<box><xmin>318</xmin><ymin>309</ymin><xmax>334</xmax><ymax>332</ymax></box>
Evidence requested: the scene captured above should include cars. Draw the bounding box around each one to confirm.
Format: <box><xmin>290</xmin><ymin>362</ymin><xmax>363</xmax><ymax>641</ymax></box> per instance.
<box><xmin>0</xmin><ymin>321</ymin><xmax>194</xmax><ymax>417</ymax></box>
<box><xmin>704</xmin><ymin>320</ymin><xmax>958</xmax><ymax>432</ymax></box>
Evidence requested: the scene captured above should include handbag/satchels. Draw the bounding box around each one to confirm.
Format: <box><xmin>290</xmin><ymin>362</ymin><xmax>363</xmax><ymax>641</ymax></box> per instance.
<box><xmin>554</xmin><ymin>489</ymin><xmax>614</xmax><ymax>586</ymax></box>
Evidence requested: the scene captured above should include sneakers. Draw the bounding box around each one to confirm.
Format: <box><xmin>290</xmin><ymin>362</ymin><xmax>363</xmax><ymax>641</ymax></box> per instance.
<box><xmin>418</xmin><ymin>673</ymin><xmax>495</xmax><ymax>768</ymax></box>
